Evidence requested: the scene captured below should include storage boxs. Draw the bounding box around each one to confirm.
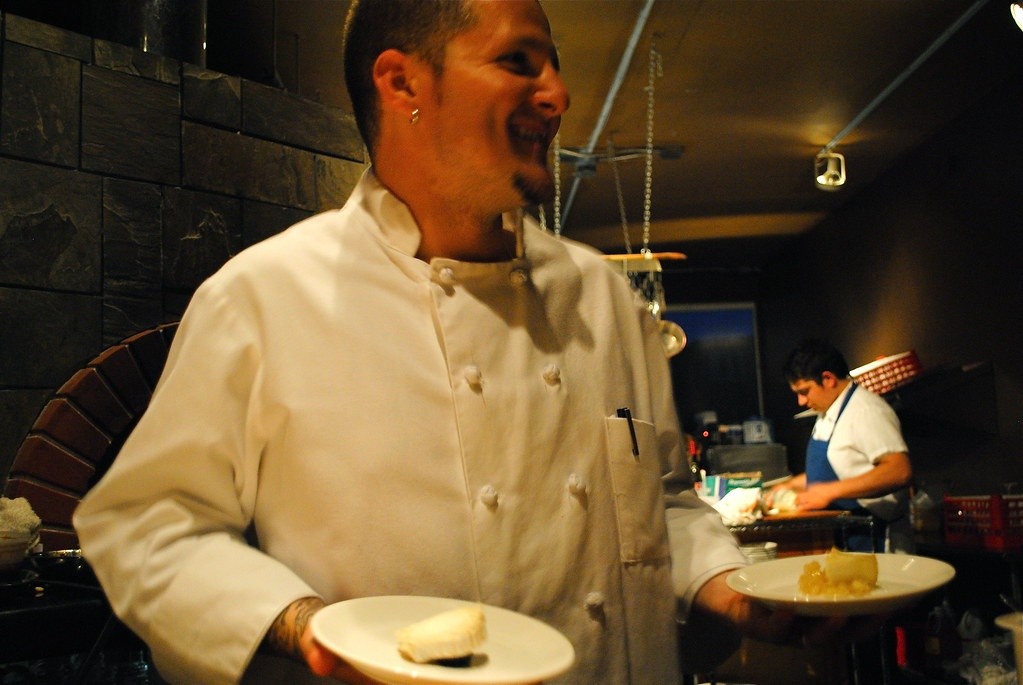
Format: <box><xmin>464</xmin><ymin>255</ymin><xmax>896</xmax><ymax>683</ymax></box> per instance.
<box><xmin>942</xmin><ymin>492</ymin><xmax>1023</xmax><ymax>554</ymax></box>
<box><xmin>847</xmin><ymin>351</ymin><xmax>924</xmax><ymax>396</ymax></box>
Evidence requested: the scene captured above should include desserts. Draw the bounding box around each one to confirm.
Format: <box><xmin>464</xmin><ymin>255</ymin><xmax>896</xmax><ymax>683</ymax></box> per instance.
<box><xmin>395</xmin><ymin>607</ymin><xmax>487</xmax><ymax>662</ymax></box>
<box><xmin>822</xmin><ymin>546</ymin><xmax>879</xmax><ymax>588</ymax></box>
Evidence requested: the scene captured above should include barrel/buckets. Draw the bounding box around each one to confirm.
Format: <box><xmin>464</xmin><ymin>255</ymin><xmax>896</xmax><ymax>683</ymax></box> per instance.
<box><xmin>738</xmin><ymin>542</ymin><xmax>778</xmax><ymax>567</ymax></box>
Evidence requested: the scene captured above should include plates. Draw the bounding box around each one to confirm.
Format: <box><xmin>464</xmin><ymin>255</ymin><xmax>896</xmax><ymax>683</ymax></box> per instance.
<box><xmin>309</xmin><ymin>594</ymin><xmax>575</xmax><ymax>685</ymax></box>
<box><xmin>725</xmin><ymin>554</ymin><xmax>955</xmax><ymax>616</ymax></box>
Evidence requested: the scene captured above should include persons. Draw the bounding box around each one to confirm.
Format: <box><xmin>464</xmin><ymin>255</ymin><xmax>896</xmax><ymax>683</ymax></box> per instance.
<box><xmin>763</xmin><ymin>340</ymin><xmax>912</xmax><ymax>685</ymax></box>
<box><xmin>73</xmin><ymin>0</ymin><xmax>889</xmax><ymax>684</ymax></box>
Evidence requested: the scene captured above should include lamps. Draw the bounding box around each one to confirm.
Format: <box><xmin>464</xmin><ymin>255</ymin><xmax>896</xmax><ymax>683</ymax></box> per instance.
<box><xmin>813</xmin><ymin>153</ymin><xmax>846</xmax><ymax>192</ymax></box>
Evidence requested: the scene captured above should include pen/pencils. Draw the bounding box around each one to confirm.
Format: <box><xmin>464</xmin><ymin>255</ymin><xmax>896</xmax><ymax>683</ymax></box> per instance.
<box><xmin>617</xmin><ymin>406</ymin><xmax>639</xmax><ymax>456</ymax></box>
